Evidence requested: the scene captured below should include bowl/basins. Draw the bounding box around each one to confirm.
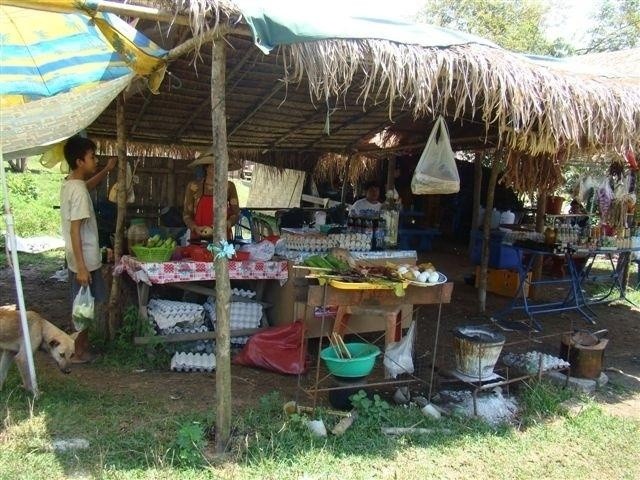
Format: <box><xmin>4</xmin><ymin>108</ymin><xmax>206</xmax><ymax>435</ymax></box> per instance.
<box><xmin>318</xmin><ymin>342</ymin><xmax>383</xmax><ymax>379</ymax></box>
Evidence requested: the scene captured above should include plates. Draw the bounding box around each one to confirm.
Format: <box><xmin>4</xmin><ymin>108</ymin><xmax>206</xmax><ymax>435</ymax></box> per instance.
<box><xmin>400</xmin><ymin>268</ymin><xmax>449</xmax><ymax>289</ymax></box>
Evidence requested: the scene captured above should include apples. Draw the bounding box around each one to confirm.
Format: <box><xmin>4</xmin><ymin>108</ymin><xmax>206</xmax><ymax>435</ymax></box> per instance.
<box><xmin>202</xmin><ymin>227</ymin><xmax>214</xmax><ymax>238</ymax></box>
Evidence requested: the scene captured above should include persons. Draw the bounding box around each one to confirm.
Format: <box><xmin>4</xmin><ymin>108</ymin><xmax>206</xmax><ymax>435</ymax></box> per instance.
<box><xmin>60</xmin><ymin>136</ymin><xmax>119</xmax><ymax>363</ymax></box>
<box><xmin>180</xmin><ymin>159</ymin><xmax>241</xmax><ymax>245</ymax></box>
<box><xmin>347</xmin><ymin>183</ymin><xmax>386</xmax><ymax>217</ymax></box>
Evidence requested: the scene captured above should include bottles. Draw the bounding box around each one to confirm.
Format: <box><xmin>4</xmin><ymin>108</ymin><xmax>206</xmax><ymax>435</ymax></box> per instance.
<box><xmin>346</xmin><ymin>207</ymin><xmax>387</xmax><ymax>250</ymax></box>
<box><xmin>553</xmin><ymin>222</ymin><xmax>631</xmax><ymax>250</ymax></box>
<box><xmin>379</xmin><ymin>190</ymin><xmax>400</xmax><ymax>249</ymax></box>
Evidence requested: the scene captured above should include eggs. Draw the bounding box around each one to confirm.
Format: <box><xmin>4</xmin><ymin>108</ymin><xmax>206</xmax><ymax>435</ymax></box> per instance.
<box><xmin>283</xmin><ymin>232</ymin><xmax>371</xmax><ymax>249</ymax></box>
<box><xmin>396</xmin><ymin>263</ymin><xmax>440</xmax><ymax>283</ymax></box>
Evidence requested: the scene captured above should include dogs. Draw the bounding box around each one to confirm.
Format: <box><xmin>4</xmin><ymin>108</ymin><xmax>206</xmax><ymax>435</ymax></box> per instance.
<box><xmin>0</xmin><ymin>309</ymin><xmax>82</xmax><ymax>395</ymax></box>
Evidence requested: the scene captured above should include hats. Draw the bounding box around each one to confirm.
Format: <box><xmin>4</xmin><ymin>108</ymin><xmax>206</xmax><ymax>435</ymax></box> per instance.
<box><xmin>186</xmin><ymin>145</ymin><xmax>239</xmax><ymax>171</ymax></box>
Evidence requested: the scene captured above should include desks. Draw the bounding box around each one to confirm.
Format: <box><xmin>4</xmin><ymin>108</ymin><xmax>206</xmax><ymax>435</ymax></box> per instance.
<box><xmin>122</xmin><ymin>255</ymin><xmax>287</xmax><ymax>360</ymax></box>
<box><xmin>492</xmin><ymin>243</ymin><xmax>640</xmax><ymax>330</ymax></box>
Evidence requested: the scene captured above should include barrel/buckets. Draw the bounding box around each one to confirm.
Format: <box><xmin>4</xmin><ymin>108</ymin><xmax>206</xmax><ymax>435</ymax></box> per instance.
<box><xmin>453</xmin><ymin>323</ymin><xmax>505</xmax><ymax>380</ymax></box>
<box><xmin>314</xmin><ymin>210</ymin><xmax>326</xmax><ymax>225</ymax></box>
<box><xmin>489</xmin><ymin>208</ymin><xmax>501</xmax><ymax>230</ymax></box>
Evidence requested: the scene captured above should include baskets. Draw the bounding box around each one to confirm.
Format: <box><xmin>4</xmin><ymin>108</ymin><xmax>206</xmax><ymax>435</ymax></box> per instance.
<box><xmin>130</xmin><ymin>244</ymin><xmax>174</xmax><ymax>262</ymax></box>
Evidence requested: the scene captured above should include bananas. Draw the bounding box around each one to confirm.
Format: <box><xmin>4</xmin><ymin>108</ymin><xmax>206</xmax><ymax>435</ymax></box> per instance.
<box><xmin>73</xmin><ymin>304</ymin><xmax>94</xmax><ymax>322</ymax></box>
<box><xmin>145</xmin><ymin>234</ymin><xmax>177</xmax><ymax>249</ymax></box>
<box><xmin>300</xmin><ymin>255</ymin><xmax>348</xmax><ymax>274</ymax></box>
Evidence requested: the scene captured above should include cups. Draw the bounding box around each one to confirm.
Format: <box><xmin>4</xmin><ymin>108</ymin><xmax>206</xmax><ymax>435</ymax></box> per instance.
<box><xmin>314</xmin><ymin>210</ymin><xmax>328</xmax><ymax>234</ymax></box>
